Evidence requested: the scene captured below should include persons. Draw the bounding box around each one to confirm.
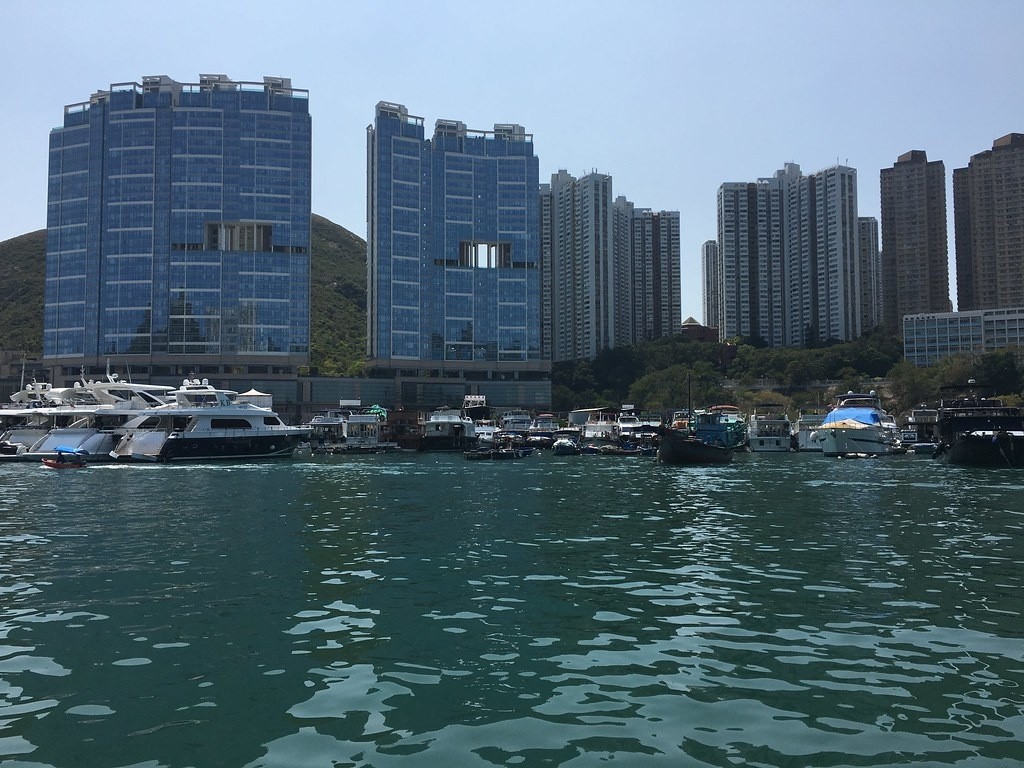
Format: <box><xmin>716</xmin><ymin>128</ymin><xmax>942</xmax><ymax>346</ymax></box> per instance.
<box><xmin>499</xmin><ymin>417</ymin><xmax>504</xmax><ymax>429</ymax></box>
<box><xmin>496</xmin><ymin>438</ymin><xmax>512</xmax><ymax>449</ymax></box>
<box><xmin>765</xmin><ymin>410</ymin><xmax>772</xmax><ymax>419</ymax></box>
<box><xmin>339</xmin><ymin>432</ymin><xmax>346</xmax><ymax>444</ymax></box>
<box><xmin>827</xmin><ymin>402</ymin><xmax>833</xmax><ymax>412</ymax></box>
<box><xmin>601</xmin><ymin>431</ymin><xmax>610</xmax><ymax>438</ymax></box>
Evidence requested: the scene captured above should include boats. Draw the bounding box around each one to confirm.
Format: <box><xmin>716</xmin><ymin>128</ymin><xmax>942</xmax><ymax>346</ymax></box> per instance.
<box><xmin>657</xmin><ymin>389</ymin><xmax>1024</xmax><ymax>467</ymax></box>
<box><xmin>1</xmin><ymin>380</ymin><xmax>316</xmax><ymax>469</ymax></box>
<box><xmin>300</xmin><ymin>400</ymin><xmax>659</xmax><ymax>461</ymax></box>
